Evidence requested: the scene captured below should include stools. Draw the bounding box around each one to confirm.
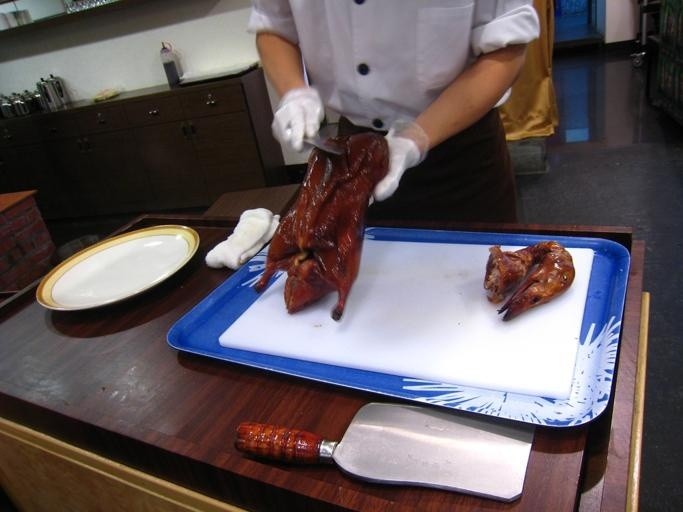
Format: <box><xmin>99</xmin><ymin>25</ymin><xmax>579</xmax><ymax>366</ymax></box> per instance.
<box><xmin>202</xmin><ymin>183</ymin><xmax>300</xmax><ymax>218</ymax></box>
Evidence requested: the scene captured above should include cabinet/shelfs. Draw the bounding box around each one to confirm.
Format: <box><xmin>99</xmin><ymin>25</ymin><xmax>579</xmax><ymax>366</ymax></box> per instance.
<box><xmin>0</xmin><ymin>66</ymin><xmax>285</xmax><ymax>220</ymax></box>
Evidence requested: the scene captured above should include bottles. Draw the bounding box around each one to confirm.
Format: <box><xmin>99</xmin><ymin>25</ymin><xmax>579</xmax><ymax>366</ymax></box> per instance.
<box><xmin>160</xmin><ymin>42</ymin><xmax>180</xmax><ymax>86</ymax></box>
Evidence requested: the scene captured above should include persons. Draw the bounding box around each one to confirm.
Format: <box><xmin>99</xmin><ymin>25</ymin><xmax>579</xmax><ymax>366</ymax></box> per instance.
<box><xmin>244</xmin><ymin>1</ymin><xmax>543</xmax><ymax>230</ymax></box>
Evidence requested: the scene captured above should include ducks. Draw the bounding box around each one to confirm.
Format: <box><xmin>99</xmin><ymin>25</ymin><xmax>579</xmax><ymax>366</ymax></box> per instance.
<box><xmin>252</xmin><ymin>130</ymin><xmax>390</xmax><ymax>322</ymax></box>
<box><xmin>485</xmin><ymin>240</ymin><xmax>574</xmax><ymax>324</ymax></box>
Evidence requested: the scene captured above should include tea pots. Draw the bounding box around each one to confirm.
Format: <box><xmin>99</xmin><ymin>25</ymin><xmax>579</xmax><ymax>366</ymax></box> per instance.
<box><xmin>37</xmin><ymin>74</ymin><xmax>71</xmax><ymax>111</ymax></box>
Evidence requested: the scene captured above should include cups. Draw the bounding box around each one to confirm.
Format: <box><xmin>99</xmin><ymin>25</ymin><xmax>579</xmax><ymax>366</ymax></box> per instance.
<box><xmin>0</xmin><ymin>92</ymin><xmax>45</xmax><ymax>118</ymax></box>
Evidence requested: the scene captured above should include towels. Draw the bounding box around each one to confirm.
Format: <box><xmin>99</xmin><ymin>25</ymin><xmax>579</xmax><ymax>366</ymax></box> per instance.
<box><xmin>205</xmin><ymin>208</ymin><xmax>281</xmax><ymax>270</ymax></box>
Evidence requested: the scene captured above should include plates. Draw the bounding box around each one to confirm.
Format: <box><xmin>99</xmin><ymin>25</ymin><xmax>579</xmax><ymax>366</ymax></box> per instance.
<box><xmin>36</xmin><ymin>225</ymin><xmax>200</xmax><ymax>311</ymax></box>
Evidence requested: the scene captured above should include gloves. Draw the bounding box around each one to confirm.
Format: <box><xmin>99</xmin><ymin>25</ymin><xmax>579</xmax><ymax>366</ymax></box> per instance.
<box><xmin>271</xmin><ymin>90</ymin><xmax>325</xmax><ymax>151</ymax></box>
<box><xmin>368</xmin><ymin>137</ymin><xmax>421</xmax><ymax>206</ymax></box>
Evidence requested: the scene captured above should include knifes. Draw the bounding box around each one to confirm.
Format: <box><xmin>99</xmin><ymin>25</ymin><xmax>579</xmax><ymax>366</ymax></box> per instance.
<box><xmin>235</xmin><ymin>402</ymin><xmax>536</xmax><ymax>502</ymax></box>
<box><xmin>303</xmin><ymin>122</ymin><xmax>346</xmax><ymax>156</ymax></box>
